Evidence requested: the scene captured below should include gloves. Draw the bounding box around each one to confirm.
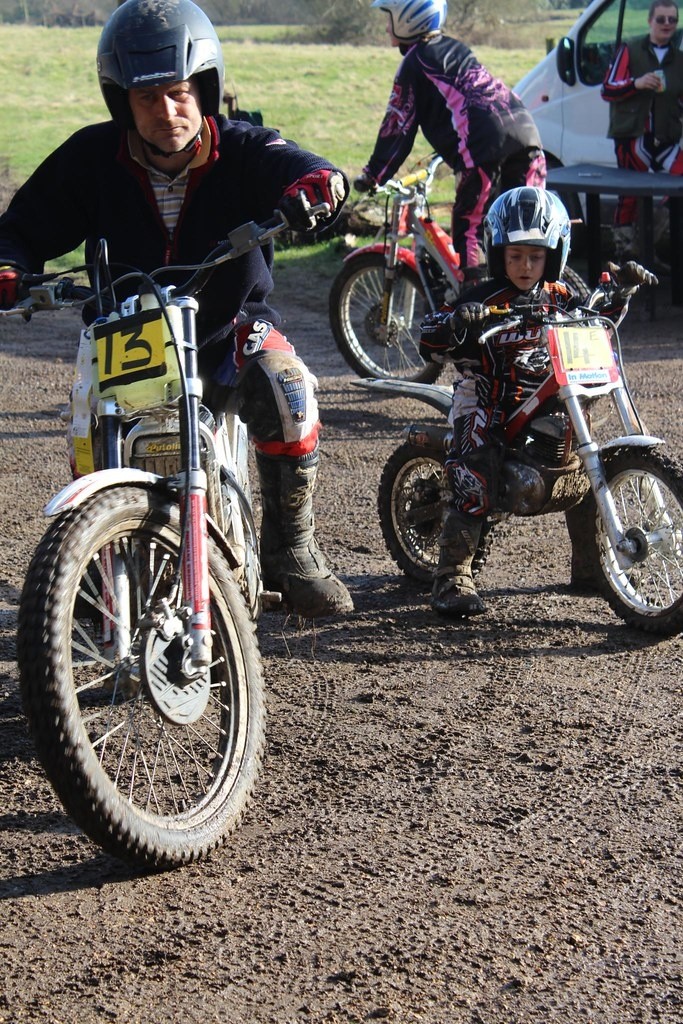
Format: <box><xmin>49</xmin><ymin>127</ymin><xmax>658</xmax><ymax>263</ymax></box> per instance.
<box><xmin>277</xmin><ymin>169</ymin><xmax>346</xmax><ymax>234</ymax></box>
<box><xmin>0</xmin><ymin>266</ymin><xmax>24</xmax><ymax>311</ymax></box>
<box><xmin>354</xmin><ymin>173</ymin><xmax>375</xmax><ymax>193</ymax></box>
<box><xmin>606</xmin><ymin>261</ymin><xmax>659</xmax><ymax>288</ymax></box>
<box><xmin>444</xmin><ymin>302</ymin><xmax>490</xmax><ymax>335</ymax></box>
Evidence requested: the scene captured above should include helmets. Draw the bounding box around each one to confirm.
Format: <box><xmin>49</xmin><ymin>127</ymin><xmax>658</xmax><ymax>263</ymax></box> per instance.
<box><xmin>483</xmin><ymin>187</ymin><xmax>571</xmax><ymax>284</ymax></box>
<box><xmin>370</xmin><ymin>0</ymin><xmax>448</xmax><ymax>44</ymax></box>
<box><xmin>96</xmin><ymin>0</ymin><xmax>224</xmax><ymax>131</ymax></box>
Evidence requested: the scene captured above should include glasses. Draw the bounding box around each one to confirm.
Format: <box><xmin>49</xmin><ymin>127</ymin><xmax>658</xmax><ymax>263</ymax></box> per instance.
<box><xmin>652</xmin><ymin>15</ymin><xmax>678</xmax><ymax>25</ymax></box>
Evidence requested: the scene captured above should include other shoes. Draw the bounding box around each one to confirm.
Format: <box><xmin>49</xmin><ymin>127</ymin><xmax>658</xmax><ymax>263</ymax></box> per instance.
<box><xmin>613</xmin><ymin>226</ymin><xmax>641</xmax><ymax>257</ymax></box>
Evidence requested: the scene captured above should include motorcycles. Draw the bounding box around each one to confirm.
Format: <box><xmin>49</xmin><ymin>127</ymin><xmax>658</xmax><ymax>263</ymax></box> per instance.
<box><xmin>375</xmin><ymin>272</ymin><xmax>683</xmax><ymax>640</ymax></box>
<box><xmin>0</xmin><ymin>183</ymin><xmax>349</xmax><ymax>877</ymax></box>
<box><xmin>330</xmin><ymin>145</ymin><xmax>606</xmax><ymax>414</ymax></box>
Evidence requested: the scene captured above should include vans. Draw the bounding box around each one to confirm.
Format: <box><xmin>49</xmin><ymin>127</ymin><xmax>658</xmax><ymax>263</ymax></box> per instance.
<box><xmin>508</xmin><ymin>1</ymin><xmax>682</xmax><ymax>233</ymax></box>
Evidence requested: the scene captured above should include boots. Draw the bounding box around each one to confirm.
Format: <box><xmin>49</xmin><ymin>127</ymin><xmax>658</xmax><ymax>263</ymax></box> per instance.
<box><xmin>255</xmin><ymin>436</ymin><xmax>354</xmax><ymax>618</ymax></box>
<box><xmin>565</xmin><ymin>499</ymin><xmax>644</xmax><ymax>591</ymax></box>
<box><xmin>75</xmin><ymin>560</ymin><xmax>106</xmax><ymax>619</ymax></box>
<box><xmin>430</xmin><ymin>506</ymin><xmax>487</xmax><ymax>615</ymax></box>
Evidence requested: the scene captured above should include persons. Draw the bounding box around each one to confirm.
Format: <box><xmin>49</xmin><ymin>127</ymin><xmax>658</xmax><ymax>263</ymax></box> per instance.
<box><xmin>355</xmin><ymin>1</ymin><xmax>548</xmax><ymax>287</ymax></box>
<box><xmin>600</xmin><ymin>1</ymin><xmax>682</xmax><ymax>275</ymax></box>
<box><xmin>2</xmin><ymin>1</ymin><xmax>352</xmax><ymax>619</ymax></box>
<box><xmin>408</xmin><ymin>188</ymin><xmax>659</xmax><ymax>622</ymax></box>
